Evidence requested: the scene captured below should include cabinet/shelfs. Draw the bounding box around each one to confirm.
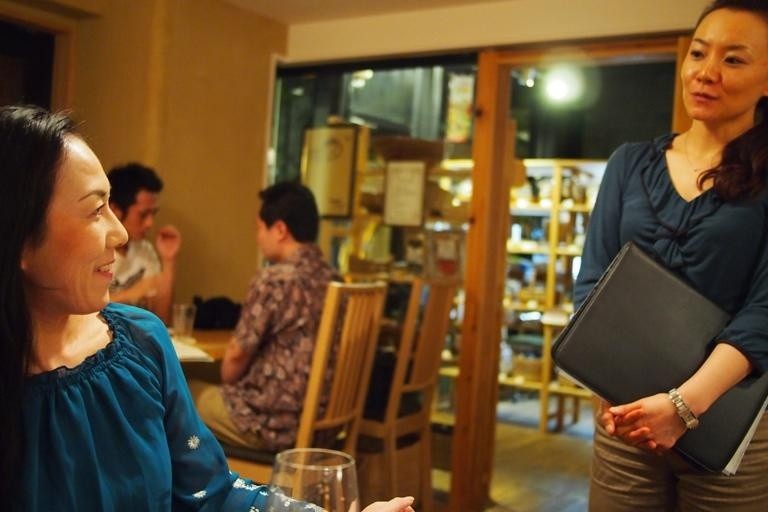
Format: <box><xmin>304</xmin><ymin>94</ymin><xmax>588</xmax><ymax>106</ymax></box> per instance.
<box><xmin>431</xmin><ymin>156</ymin><xmax>594</xmax><ymax>434</ymax></box>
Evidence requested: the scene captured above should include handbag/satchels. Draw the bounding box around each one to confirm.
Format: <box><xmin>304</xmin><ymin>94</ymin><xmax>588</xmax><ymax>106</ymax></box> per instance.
<box><xmin>357</xmin><ymin>353</ymin><xmax>420</xmax><ymax>452</ymax></box>
<box><xmin>193</xmin><ymin>296</ymin><xmax>240</xmax><ymax>330</ymax></box>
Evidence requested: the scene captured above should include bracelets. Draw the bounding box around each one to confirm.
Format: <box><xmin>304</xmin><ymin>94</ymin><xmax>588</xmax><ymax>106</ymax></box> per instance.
<box><xmin>668</xmin><ymin>388</ymin><xmax>700</xmax><ymax>431</ymax></box>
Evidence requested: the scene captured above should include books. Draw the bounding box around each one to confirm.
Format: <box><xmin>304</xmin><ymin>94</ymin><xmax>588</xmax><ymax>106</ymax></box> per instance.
<box><xmin>551</xmin><ymin>241</ymin><xmax>768</xmax><ymax>476</ymax></box>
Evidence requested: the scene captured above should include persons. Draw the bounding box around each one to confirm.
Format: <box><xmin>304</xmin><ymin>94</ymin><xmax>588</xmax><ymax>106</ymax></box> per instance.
<box><xmin>189</xmin><ymin>182</ymin><xmax>349</xmax><ymax>456</ymax></box>
<box><xmin>574</xmin><ymin>0</ymin><xmax>768</xmax><ymax>512</ymax></box>
<box><xmin>0</xmin><ymin>104</ymin><xmax>415</xmax><ymax>512</ymax></box>
<box><xmin>106</xmin><ymin>163</ymin><xmax>182</xmax><ymax>328</ymax></box>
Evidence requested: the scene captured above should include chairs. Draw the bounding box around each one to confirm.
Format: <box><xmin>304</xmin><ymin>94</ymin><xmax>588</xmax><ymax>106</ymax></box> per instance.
<box><xmin>325</xmin><ymin>274</ymin><xmax>457</xmax><ymax>510</ymax></box>
<box><xmin>224</xmin><ymin>273</ymin><xmax>389</xmax><ymax>509</ymax></box>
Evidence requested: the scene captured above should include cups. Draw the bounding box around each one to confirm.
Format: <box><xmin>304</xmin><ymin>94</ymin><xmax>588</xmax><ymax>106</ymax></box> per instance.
<box><xmin>172</xmin><ymin>301</ymin><xmax>197</xmax><ymax>345</ymax></box>
<box><xmin>262</xmin><ymin>448</ymin><xmax>360</xmax><ymax>512</ymax></box>
